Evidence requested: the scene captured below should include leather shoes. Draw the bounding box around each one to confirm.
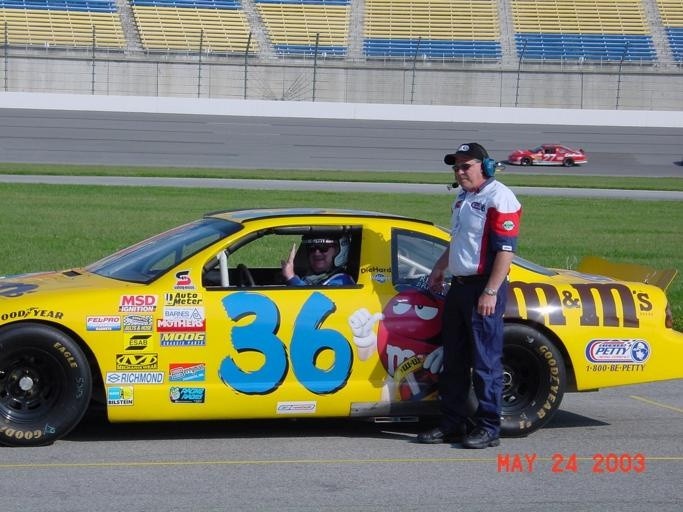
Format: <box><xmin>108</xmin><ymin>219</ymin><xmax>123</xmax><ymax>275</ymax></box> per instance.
<box><xmin>417</xmin><ymin>426</ymin><xmax>457</xmax><ymax>444</ymax></box>
<box><xmin>463</xmin><ymin>428</ymin><xmax>499</xmax><ymax>448</ymax></box>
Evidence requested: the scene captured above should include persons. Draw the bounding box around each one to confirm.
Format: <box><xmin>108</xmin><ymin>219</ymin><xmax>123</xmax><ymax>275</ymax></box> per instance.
<box><xmin>279</xmin><ymin>233</ymin><xmax>355</xmax><ymax>285</ymax></box>
<box><xmin>419</xmin><ymin>142</ymin><xmax>524</xmax><ymax>449</ymax></box>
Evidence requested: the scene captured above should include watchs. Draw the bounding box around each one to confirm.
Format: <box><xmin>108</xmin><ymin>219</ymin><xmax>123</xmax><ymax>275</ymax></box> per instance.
<box><xmin>484</xmin><ymin>288</ymin><xmax>497</xmax><ymax>296</ymax></box>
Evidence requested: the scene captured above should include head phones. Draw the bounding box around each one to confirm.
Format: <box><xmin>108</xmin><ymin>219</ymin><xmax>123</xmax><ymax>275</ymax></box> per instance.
<box><xmin>453</xmin><ymin>158</ymin><xmax>506</xmax><ymax>177</ymax></box>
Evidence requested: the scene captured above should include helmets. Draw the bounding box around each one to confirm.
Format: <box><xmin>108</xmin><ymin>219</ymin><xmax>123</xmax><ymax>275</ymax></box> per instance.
<box><xmin>301</xmin><ymin>235</ymin><xmax>350</xmax><ymax>267</ymax></box>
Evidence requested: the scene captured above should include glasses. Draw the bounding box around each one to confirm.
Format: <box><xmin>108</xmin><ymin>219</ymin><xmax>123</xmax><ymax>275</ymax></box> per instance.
<box><xmin>306</xmin><ymin>244</ymin><xmax>328</xmax><ymax>254</ymax></box>
<box><xmin>451</xmin><ymin>161</ymin><xmax>480</xmax><ymax>171</ymax></box>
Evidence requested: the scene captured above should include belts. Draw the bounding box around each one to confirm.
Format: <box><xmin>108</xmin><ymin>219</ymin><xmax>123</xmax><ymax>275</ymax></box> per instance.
<box><xmin>452</xmin><ymin>275</ymin><xmax>473</xmax><ymax>284</ymax></box>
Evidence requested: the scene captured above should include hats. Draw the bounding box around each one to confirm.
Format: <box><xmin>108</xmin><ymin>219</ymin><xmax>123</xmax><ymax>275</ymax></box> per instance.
<box><xmin>444</xmin><ymin>143</ymin><xmax>489</xmax><ymax>165</ymax></box>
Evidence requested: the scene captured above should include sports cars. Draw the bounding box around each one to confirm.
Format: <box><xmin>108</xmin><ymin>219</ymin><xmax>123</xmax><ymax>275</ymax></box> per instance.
<box><xmin>508</xmin><ymin>144</ymin><xmax>588</xmax><ymax>167</ymax></box>
<box><xmin>0</xmin><ymin>207</ymin><xmax>683</xmax><ymax>447</ymax></box>
